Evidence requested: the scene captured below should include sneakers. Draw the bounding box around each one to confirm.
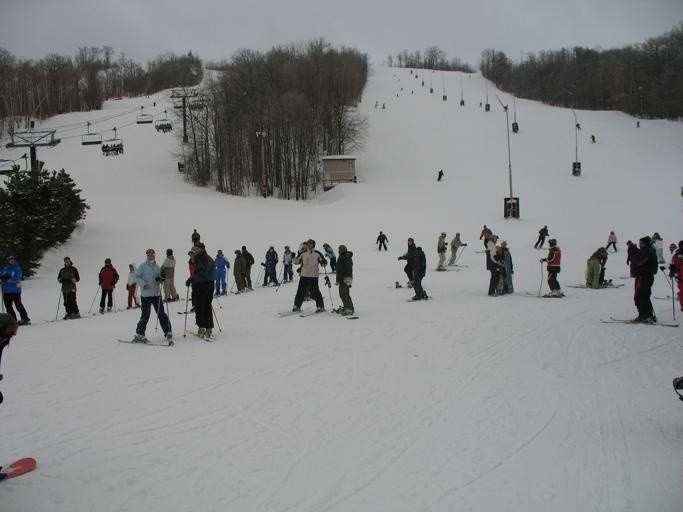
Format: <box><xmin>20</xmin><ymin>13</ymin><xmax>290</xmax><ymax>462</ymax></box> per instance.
<box><xmin>214</xmin><ymin>280</ymin><xmax>293</xmax><ymax>297</ymax></box>
<box><xmin>405</xmin><ymin>279</ymin><xmax>660</xmax><ymax>323</ymax></box>
<box><xmin>205</xmin><ymin>328</ymin><xmax>212</xmax><ymax>338</ymax></box>
<box><xmin>135</xmin><ymin>334</ymin><xmax>147</xmax><ymax>341</ymax></box>
<box><xmin>62</xmin><ymin>295</ymin><xmax>179</xmax><ymax>321</ymax></box>
<box><xmin>166</xmin><ymin>332</ymin><xmax>172</xmax><ymax>341</ymax></box>
<box><xmin>198</xmin><ymin>327</ymin><xmax>206</xmax><ymax>338</ymax></box>
<box><xmin>18</xmin><ymin>319</ymin><xmax>31</xmax><ymax>325</ymax></box>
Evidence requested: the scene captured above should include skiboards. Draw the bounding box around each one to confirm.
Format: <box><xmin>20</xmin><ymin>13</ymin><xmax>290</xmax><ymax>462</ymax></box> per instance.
<box><xmin>601</xmin><ymin>294</ymin><xmax>681</xmax><ymax>330</ymax></box>
<box><xmin>118</xmin><ymin>329</ymin><xmax>217</xmax><ymax>345</ymax></box>
<box><xmin>18</xmin><ymin>297</ymin><xmax>184</xmax><ymax>327</ymax></box>
<box><xmin>278</xmin><ymin>304</ymin><xmax>335</xmax><ymax>317</ymax></box>
<box><xmin>426</xmin><ymin>250</ymin><xmax>487</xmax><ymax>272</ymax></box>
<box><xmin>0</xmin><ymin>457</ymin><xmax>36</xmax><ymax>480</ymax></box>
<box><xmin>319</xmin><ymin>272</ymin><xmax>337</xmax><ymax>274</ymax></box>
<box><xmin>213</xmin><ymin>279</ymin><xmax>294</xmax><ymax>299</ymax></box>
<box><xmin>178</xmin><ymin>310</ymin><xmax>195</xmax><ymax>314</ymax></box>
<box><xmin>520</xmin><ymin>277</ymin><xmax>625</xmax><ymax>300</ymax></box>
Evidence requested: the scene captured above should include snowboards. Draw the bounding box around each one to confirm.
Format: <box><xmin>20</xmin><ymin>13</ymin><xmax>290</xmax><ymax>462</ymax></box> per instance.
<box><xmin>331</xmin><ymin>306</ymin><xmax>358</xmax><ymax>319</ymax></box>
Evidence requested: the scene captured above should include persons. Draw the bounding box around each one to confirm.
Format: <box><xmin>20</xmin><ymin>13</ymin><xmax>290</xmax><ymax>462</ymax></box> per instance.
<box><xmin>291</xmin><ymin>238</ymin><xmax>355</xmax><ymax>316</ymax></box>
<box><xmin>587</xmin><ymin>228</ymin><xmax>683</xmax><ymax>327</ymax></box>
<box><xmin>1</xmin><ymin>256</ymin><xmax>31</xmax><ymax>324</ymax></box>
<box><xmin>437</xmin><ymin>169</ymin><xmax>444</xmax><ymax>182</ymax></box>
<box><xmin>135</xmin><ymin>229</ymin><xmax>217</xmax><ymax>343</ymax></box>
<box><xmin>126</xmin><ymin>264</ymin><xmax>140</xmax><ymax>310</ymax></box>
<box><xmin>0</xmin><ymin>310</ymin><xmax>18</xmax><ymax>483</ymax></box>
<box><xmin>57</xmin><ymin>256</ymin><xmax>80</xmax><ymax>320</ymax></box>
<box><xmin>376</xmin><ymin>232</ymin><xmax>388</xmax><ymax>252</ymax></box>
<box><xmin>155</xmin><ymin>123</ymin><xmax>173</xmax><ymax>134</ymax></box>
<box><xmin>100</xmin><ymin>142</ymin><xmax>125</xmax><ymax>157</ymax></box>
<box><xmin>215</xmin><ymin>245</ymin><xmax>293</xmax><ymax>297</ymax></box>
<box><xmin>398</xmin><ymin>231</ymin><xmax>467</xmax><ymax>299</ymax></box>
<box><xmin>480</xmin><ymin>224</ymin><xmax>562</xmax><ymax>297</ymax></box>
<box><xmin>99</xmin><ymin>258</ymin><xmax>119</xmax><ymax>314</ymax></box>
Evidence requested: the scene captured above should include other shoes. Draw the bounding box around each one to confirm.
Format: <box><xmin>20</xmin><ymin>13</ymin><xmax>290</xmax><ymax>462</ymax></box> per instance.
<box><xmin>336</xmin><ymin>308</ymin><xmax>354</xmax><ymax>316</ymax></box>
<box><xmin>317</xmin><ymin>307</ymin><xmax>324</xmax><ymax>313</ymax></box>
<box><xmin>293</xmin><ymin>305</ymin><xmax>300</xmax><ymax>311</ymax></box>
